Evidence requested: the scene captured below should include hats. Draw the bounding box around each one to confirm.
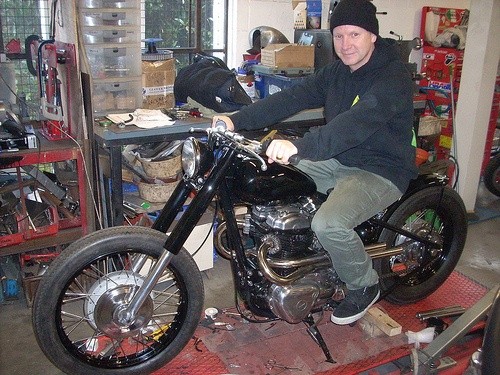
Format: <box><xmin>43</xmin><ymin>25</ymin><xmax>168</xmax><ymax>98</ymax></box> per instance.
<box><xmin>329</xmin><ymin>0</ymin><xmax>379</xmax><ymax>39</ymax></box>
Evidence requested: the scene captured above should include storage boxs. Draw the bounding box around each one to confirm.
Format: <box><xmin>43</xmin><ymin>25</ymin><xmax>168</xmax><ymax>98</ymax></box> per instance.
<box><xmin>260</xmin><ymin>43</ymin><xmax>315</xmax><ymax>68</ymax></box>
<box><xmin>114</xmin><ymin>93</ymin><xmax>137</xmax><ymax>110</ymax></box>
<box><xmin>255</xmin><ymin>76</ymin><xmax>307</xmax><ymax>99</ymax></box>
<box><xmin>292</xmin><ymin>0</ymin><xmax>322</xmax><ymax>30</ymax></box>
<box><xmin>294</xmin><ymin>28</ymin><xmax>333</xmax><ymax>68</ymax></box>
<box><xmin>237</xmin><ymin>74</ymin><xmax>254</xmax><ymax>84</ymax></box>
<box><xmin>419</xmin><ymin>5</ymin><xmax>500</xmax><ymax>186</ymax></box>
<box><xmin>142</xmin><ymin>57</ymin><xmax>176</xmax><ymax>111</ymax></box>
<box><xmin>91</xmin><ymin>91</ymin><xmax>116</xmax><ymax>112</ymax></box>
<box><xmin>134</xmin><ymin>221</ymin><xmax>214</xmax><ymax>283</ymax></box>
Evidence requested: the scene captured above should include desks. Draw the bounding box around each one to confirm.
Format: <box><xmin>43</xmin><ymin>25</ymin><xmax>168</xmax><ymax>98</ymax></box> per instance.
<box><xmin>92</xmin><ymin>100</ymin><xmax>425</xmax><ymax>272</ymax></box>
<box><xmin>0</xmin><ymin>128</ymin><xmax>97</xmax><ymax>287</ymax></box>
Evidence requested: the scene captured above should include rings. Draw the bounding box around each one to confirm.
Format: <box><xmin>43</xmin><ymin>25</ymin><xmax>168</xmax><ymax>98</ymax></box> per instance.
<box><xmin>276</xmin><ymin>156</ymin><xmax>282</xmax><ymax>160</ymax></box>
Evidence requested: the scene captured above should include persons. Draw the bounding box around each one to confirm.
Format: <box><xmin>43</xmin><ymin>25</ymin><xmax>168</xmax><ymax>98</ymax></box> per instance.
<box><xmin>212</xmin><ymin>0</ymin><xmax>419</xmax><ymax>325</ymax></box>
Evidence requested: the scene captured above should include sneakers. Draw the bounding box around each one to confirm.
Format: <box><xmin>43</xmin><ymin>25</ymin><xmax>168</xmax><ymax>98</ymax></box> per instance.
<box><xmin>330</xmin><ymin>281</ymin><xmax>381</xmax><ymax>325</ymax></box>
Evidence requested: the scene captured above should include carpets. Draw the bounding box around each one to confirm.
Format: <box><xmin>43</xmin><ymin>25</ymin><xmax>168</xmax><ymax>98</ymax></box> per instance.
<box><xmin>89</xmin><ymin>262</ymin><xmax>494</xmax><ymax>375</ymax></box>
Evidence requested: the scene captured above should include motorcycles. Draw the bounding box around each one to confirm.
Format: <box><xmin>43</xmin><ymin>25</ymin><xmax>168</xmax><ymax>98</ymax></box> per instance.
<box><xmin>32</xmin><ymin>113</ymin><xmax>469</xmax><ymax>375</ymax></box>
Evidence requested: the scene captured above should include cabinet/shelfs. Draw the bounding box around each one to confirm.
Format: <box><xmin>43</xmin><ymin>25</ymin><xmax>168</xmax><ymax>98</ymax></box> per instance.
<box><xmin>55</xmin><ymin>0</ymin><xmax>144</xmax><ymax>119</ymax></box>
